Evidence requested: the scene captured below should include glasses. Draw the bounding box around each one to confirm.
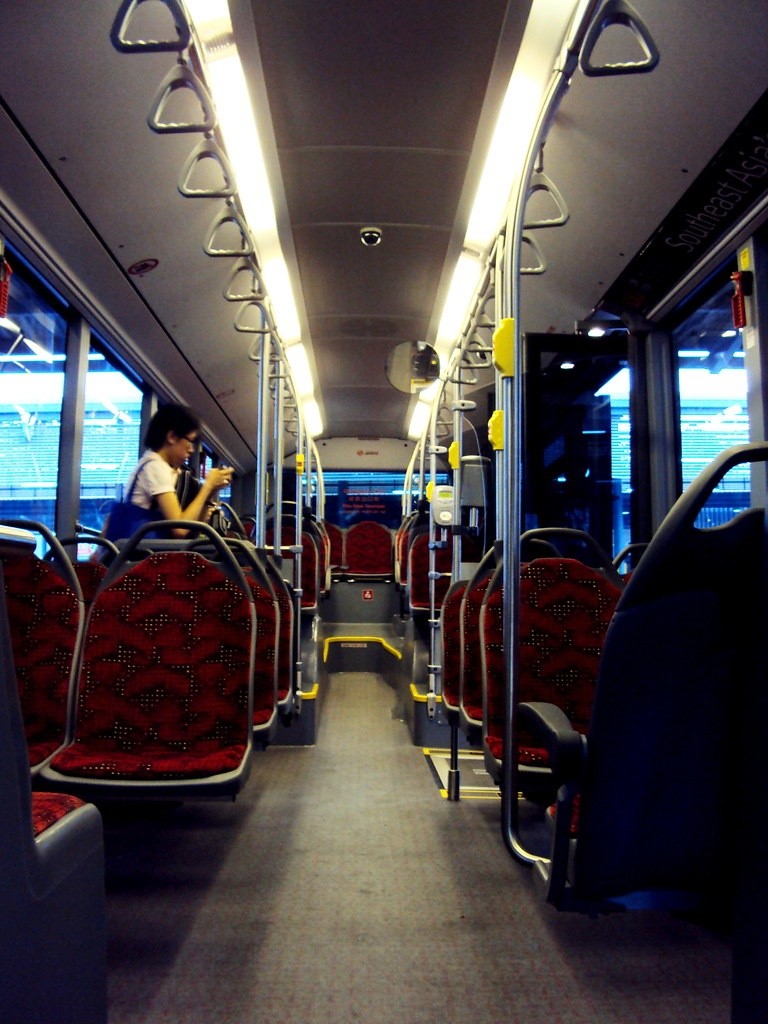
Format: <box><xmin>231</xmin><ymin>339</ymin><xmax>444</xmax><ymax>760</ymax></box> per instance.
<box><xmin>178</xmin><ymin>434</ymin><xmax>198</xmax><ymax>444</ymax></box>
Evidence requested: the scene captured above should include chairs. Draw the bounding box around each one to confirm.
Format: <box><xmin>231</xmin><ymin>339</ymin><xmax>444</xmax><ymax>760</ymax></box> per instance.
<box><xmin>1</xmin><ymin>449</ymin><xmax>768</xmax><ymax>1024</ymax></box>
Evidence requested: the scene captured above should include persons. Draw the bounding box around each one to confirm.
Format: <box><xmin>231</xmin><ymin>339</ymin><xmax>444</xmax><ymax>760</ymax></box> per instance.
<box><xmin>96</xmin><ymin>403</ymin><xmax>235</xmax><ymax>567</ymax></box>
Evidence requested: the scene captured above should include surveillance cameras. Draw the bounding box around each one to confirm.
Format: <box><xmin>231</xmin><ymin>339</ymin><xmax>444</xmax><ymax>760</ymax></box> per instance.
<box><xmin>360</xmin><ymin>227</ymin><xmax>382</xmax><ymax>246</ymax></box>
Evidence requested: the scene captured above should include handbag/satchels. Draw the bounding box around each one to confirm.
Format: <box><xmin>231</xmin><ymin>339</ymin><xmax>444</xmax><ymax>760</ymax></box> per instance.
<box><xmin>99</xmin><ymin>462</ymin><xmax>167</xmax><ymax>568</ymax></box>
